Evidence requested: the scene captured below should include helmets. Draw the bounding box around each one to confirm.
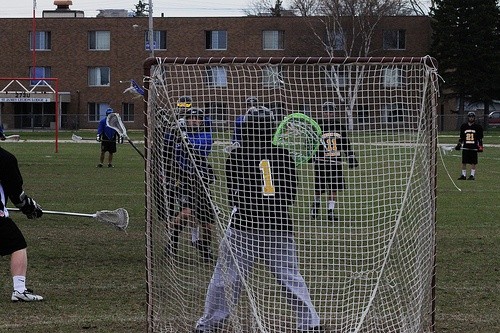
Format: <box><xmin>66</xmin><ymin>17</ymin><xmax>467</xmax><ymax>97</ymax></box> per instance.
<box><xmin>244</xmin><ymin>106</ymin><xmax>274</xmax><ymax>143</ymax></box>
<box><xmin>467</xmin><ymin>112</ymin><xmax>476</xmax><ymax>117</ymax></box>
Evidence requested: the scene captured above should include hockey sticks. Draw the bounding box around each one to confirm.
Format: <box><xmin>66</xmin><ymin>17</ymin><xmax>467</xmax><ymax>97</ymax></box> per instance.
<box><xmin>129</xmin><ymin>81</ymin><xmax>145</xmax><ymax>98</ymax></box>
<box><xmin>7</xmin><ymin>207</ymin><xmax>130</xmax><ymax>230</ymax></box>
<box><xmin>106</xmin><ymin>112</ymin><xmax>145</xmax><ymax>158</ymax></box>
<box><xmin>272</xmin><ymin>112</ymin><xmax>323</xmax><ymax>166</ymax></box>
<box><xmin>441</xmin><ymin>146</ymin><xmax>478</xmax><ymax>151</ymax></box>
<box><xmin>289</xmin><ymin>152</ymin><xmax>349</xmax><ymax>166</ymax></box>
<box><xmin>209</xmin><ymin>141</ymin><xmax>240</xmax><ymax>154</ymax></box>
<box><xmin>5</xmin><ymin>134</ymin><xmax>21</xmax><ymax>139</ymax></box>
<box><xmin>72</xmin><ymin>134</ymin><xmax>119</xmax><ymax>142</ymax></box>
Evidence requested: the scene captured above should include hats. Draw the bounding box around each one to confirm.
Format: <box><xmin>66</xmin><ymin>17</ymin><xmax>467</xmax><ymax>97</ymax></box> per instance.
<box><xmin>184</xmin><ymin>108</ymin><xmax>204</xmax><ymax>121</ymax></box>
<box><xmin>177</xmin><ymin>97</ymin><xmax>192</xmax><ymax>108</ymax></box>
<box><xmin>106</xmin><ymin>108</ymin><xmax>113</xmax><ymax>113</ymax></box>
<box><xmin>322</xmin><ymin>102</ymin><xmax>337</xmax><ymax>110</ymax></box>
<box><xmin>246</xmin><ymin>98</ymin><xmax>258</xmax><ymax>105</ymax></box>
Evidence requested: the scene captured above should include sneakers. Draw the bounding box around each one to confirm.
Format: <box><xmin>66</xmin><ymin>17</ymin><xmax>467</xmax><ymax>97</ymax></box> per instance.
<box><xmin>311</xmin><ymin>202</ymin><xmax>319</xmax><ymax>218</ymax></box>
<box><xmin>10</xmin><ymin>288</ymin><xmax>44</xmax><ymax>301</ymax></box>
<box><xmin>197</xmin><ymin>243</ymin><xmax>213</xmax><ymax>261</ymax></box>
<box><xmin>327</xmin><ymin>209</ymin><xmax>338</xmax><ymax>221</ymax></box>
<box><xmin>163</xmin><ymin>246</ymin><xmax>178</xmax><ymax>257</ymax></box>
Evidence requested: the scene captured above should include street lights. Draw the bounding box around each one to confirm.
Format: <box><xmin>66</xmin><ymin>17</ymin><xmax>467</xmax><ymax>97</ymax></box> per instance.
<box><xmin>132</xmin><ymin>24</ymin><xmax>155</xmax><ymax>76</ymax></box>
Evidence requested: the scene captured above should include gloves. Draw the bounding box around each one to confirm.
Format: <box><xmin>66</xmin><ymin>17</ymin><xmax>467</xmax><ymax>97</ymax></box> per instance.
<box><xmin>15</xmin><ymin>195</ymin><xmax>43</xmax><ymax>219</ymax></box>
<box><xmin>118</xmin><ymin>137</ymin><xmax>123</xmax><ymax>144</ymax></box>
<box><xmin>96</xmin><ymin>134</ymin><xmax>101</xmax><ymax>141</ymax></box>
<box><xmin>478</xmin><ymin>145</ymin><xmax>483</xmax><ymax>152</ymax></box>
<box><xmin>455</xmin><ymin>143</ymin><xmax>461</xmax><ymax>150</ymax></box>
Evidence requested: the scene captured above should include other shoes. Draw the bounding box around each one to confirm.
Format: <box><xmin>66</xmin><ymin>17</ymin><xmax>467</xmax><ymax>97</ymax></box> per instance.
<box><xmin>458</xmin><ymin>175</ymin><xmax>466</xmax><ymax>180</ymax></box>
<box><xmin>467</xmin><ymin>175</ymin><xmax>474</xmax><ymax>180</ymax></box>
<box><xmin>96</xmin><ymin>164</ymin><xmax>103</xmax><ymax>168</ymax></box>
<box><xmin>107</xmin><ymin>164</ymin><xmax>115</xmax><ymax>168</ymax></box>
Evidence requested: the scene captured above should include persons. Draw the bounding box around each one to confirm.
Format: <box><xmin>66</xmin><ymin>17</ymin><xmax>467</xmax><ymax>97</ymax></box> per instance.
<box><xmin>97</xmin><ymin>95</ymin><xmax>359</xmax><ymax>333</ymax></box>
<box><xmin>455</xmin><ymin>112</ymin><xmax>484</xmax><ymax>180</ymax></box>
<box><xmin>0</xmin><ymin>124</ymin><xmax>44</xmax><ymax>303</ymax></box>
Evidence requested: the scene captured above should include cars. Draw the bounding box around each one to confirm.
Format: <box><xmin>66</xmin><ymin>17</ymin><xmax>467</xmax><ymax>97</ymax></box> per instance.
<box><xmin>475</xmin><ymin>110</ymin><xmax>500</xmax><ymax>131</ymax></box>
